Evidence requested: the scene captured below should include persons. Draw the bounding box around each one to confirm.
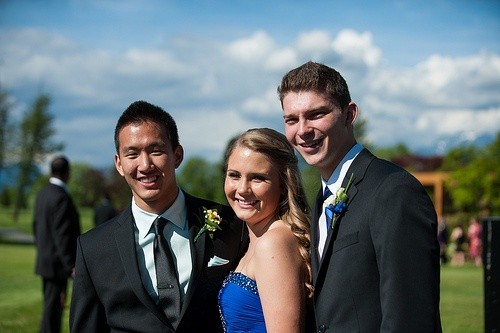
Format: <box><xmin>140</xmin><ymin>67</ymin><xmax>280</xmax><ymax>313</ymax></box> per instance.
<box><xmin>28</xmin><ymin>155</ymin><xmax>83</xmax><ymax>333</ymax></box>
<box><xmin>438</xmin><ymin>216</ymin><xmax>482</xmax><ymax>267</ymax></box>
<box><xmin>276</xmin><ymin>61</ymin><xmax>442</xmax><ymax>333</ymax></box>
<box><xmin>208</xmin><ymin>127</ymin><xmax>313</xmax><ymax>332</ymax></box>
<box><xmin>92</xmin><ymin>187</ymin><xmax>119</xmax><ymax>226</ymax></box>
<box><xmin>70</xmin><ymin>100</ymin><xmax>250</xmax><ymax>333</ymax></box>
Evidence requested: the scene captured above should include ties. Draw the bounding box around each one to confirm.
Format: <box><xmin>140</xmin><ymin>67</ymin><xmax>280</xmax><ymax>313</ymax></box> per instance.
<box><xmin>317</xmin><ymin>185</ymin><xmax>334</xmax><ymax>219</ymax></box>
<box><xmin>152</xmin><ymin>217</ymin><xmax>181</xmax><ymax>329</ymax></box>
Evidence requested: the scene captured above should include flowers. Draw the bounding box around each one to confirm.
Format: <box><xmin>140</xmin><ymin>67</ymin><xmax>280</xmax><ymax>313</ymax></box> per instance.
<box><xmin>193</xmin><ymin>206</ymin><xmax>222</xmax><ymax>244</ymax></box>
<box><xmin>331</xmin><ymin>173</ymin><xmax>355</xmax><ymax>230</ymax></box>
